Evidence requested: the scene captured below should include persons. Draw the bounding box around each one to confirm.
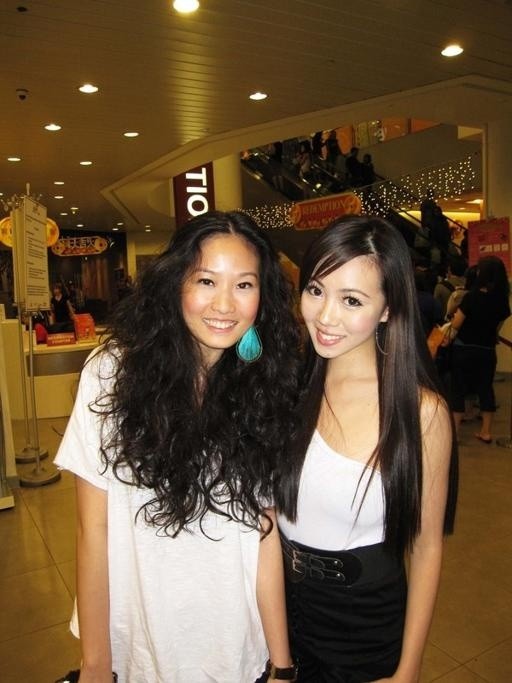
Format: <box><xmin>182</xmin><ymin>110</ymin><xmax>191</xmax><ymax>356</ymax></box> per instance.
<box><xmin>50</xmin><ymin>209</ymin><xmax>307</xmax><ymax>683</ymax></box>
<box><xmin>48</xmin><ymin>282</ymin><xmax>75</xmax><ymax>326</ymax></box>
<box><xmin>274</xmin><ymin>212</ymin><xmax>457</xmax><ymax>681</ymax></box>
<box><xmin>267</xmin><ymin>126</ymin><xmax>512</xmax><ymax>444</ymax></box>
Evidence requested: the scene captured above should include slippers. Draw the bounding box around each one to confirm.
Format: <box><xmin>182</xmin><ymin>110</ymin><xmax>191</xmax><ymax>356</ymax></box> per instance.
<box><xmin>473</xmin><ymin>430</ymin><xmax>493</xmax><ymax>444</ymax></box>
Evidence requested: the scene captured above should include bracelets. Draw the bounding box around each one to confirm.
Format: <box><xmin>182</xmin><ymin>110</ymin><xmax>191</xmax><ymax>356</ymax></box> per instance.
<box><xmin>264</xmin><ymin>658</ymin><xmax>299</xmax><ymax>680</ymax></box>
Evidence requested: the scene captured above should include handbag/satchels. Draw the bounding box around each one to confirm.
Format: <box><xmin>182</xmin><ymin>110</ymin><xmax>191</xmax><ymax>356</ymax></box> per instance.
<box><xmin>427</xmin><ymin>321</ymin><xmax>453</xmax><ymax>360</ymax></box>
